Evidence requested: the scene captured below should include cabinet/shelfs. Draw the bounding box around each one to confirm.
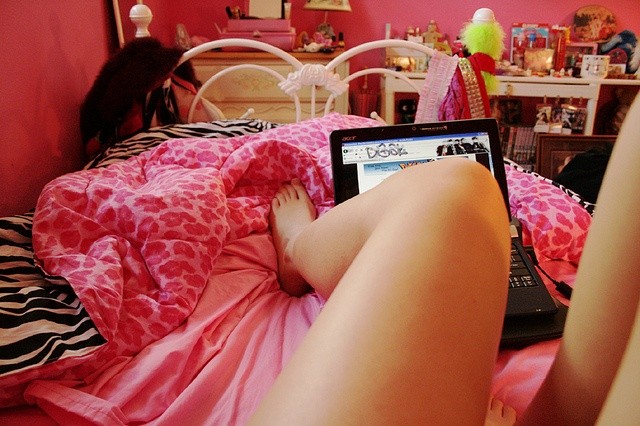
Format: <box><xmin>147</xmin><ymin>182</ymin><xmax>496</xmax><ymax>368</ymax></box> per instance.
<box><xmin>377</xmin><ymin>69</ymin><xmax>639</xmax><ymax>136</ymax></box>
<box><xmin>190</xmin><ymin>48</ymin><xmax>352</xmax><ymax>124</ymax></box>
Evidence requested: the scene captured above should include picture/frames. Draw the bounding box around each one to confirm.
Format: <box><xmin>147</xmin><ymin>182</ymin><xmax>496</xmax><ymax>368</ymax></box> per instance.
<box><xmin>538</xmin><ymin>133</ymin><xmax>616</xmax><ymax>180</ymax></box>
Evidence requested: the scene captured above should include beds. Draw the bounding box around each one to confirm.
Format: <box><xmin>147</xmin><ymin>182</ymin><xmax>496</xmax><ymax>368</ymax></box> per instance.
<box><xmin>3</xmin><ymin>38</ymin><xmax>596</xmax><ymax>425</ymax></box>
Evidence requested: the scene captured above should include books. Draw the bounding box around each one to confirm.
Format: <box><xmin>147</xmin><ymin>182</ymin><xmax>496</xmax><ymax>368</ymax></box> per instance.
<box><xmin>500</xmin><ymin>123</ymin><xmax>539</xmax><ymax>165</ymax></box>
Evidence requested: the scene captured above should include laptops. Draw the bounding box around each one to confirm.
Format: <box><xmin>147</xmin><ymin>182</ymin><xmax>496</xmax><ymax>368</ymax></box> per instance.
<box><xmin>328</xmin><ymin>117</ymin><xmax>568</xmax><ymax>344</ymax></box>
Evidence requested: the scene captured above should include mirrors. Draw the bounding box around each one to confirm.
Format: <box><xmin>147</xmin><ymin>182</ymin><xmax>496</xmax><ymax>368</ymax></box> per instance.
<box><xmin>245</xmin><ymin>0</ymin><xmax>285</xmax><ymax>20</ymax></box>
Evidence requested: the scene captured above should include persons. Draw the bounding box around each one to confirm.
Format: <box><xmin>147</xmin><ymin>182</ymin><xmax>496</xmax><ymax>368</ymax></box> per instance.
<box><xmin>249</xmin><ymin>88</ymin><xmax>639</xmax><ymax>425</ymax></box>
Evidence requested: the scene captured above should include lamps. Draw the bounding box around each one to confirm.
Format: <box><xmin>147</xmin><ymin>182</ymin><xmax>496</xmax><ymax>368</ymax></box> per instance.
<box><xmin>303</xmin><ymin>1</ymin><xmax>353</xmax><ymax>26</ymax></box>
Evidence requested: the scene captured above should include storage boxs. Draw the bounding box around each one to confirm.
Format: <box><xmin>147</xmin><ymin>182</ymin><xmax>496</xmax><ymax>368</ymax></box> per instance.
<box><xmin>218</xmin><ymin>19</ymin><xmax>296</xmax><ymax>53</ymax></box>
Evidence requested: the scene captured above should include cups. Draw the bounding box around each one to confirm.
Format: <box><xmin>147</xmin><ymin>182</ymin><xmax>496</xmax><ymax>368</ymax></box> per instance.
<box><xmin>579</xmin><ymin>54</ymin><xmax>610</xmax><ymax>82</ymax></box>
<box><xmin>523</xmin><ymin>48</ymin><xmax>555</xmax><ymax>76</ymax></box>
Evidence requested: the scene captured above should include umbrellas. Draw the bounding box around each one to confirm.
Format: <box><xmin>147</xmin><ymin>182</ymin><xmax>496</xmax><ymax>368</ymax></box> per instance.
<box><xmin>351</xmin><ymin>65</ymin><xmax>379</xmax><ymax>117</ymax></box>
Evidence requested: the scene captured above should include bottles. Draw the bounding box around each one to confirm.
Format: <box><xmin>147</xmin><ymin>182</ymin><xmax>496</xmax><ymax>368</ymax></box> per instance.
<box><xmin>527</xmin><ymin>33</ymin><xmax>536</xmax><ymax>52</ymax></box>
<box><xmin>513</xmin><ymin>28</ymin><xmax>525</xmax><ymax>70</ymax></box>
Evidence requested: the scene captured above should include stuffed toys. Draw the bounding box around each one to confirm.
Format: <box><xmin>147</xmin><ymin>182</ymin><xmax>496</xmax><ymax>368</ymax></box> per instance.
<box><xmin>462</xmin><ymin>8</ymin><xmax>505</xmax><ymax>91</ymax></box>
